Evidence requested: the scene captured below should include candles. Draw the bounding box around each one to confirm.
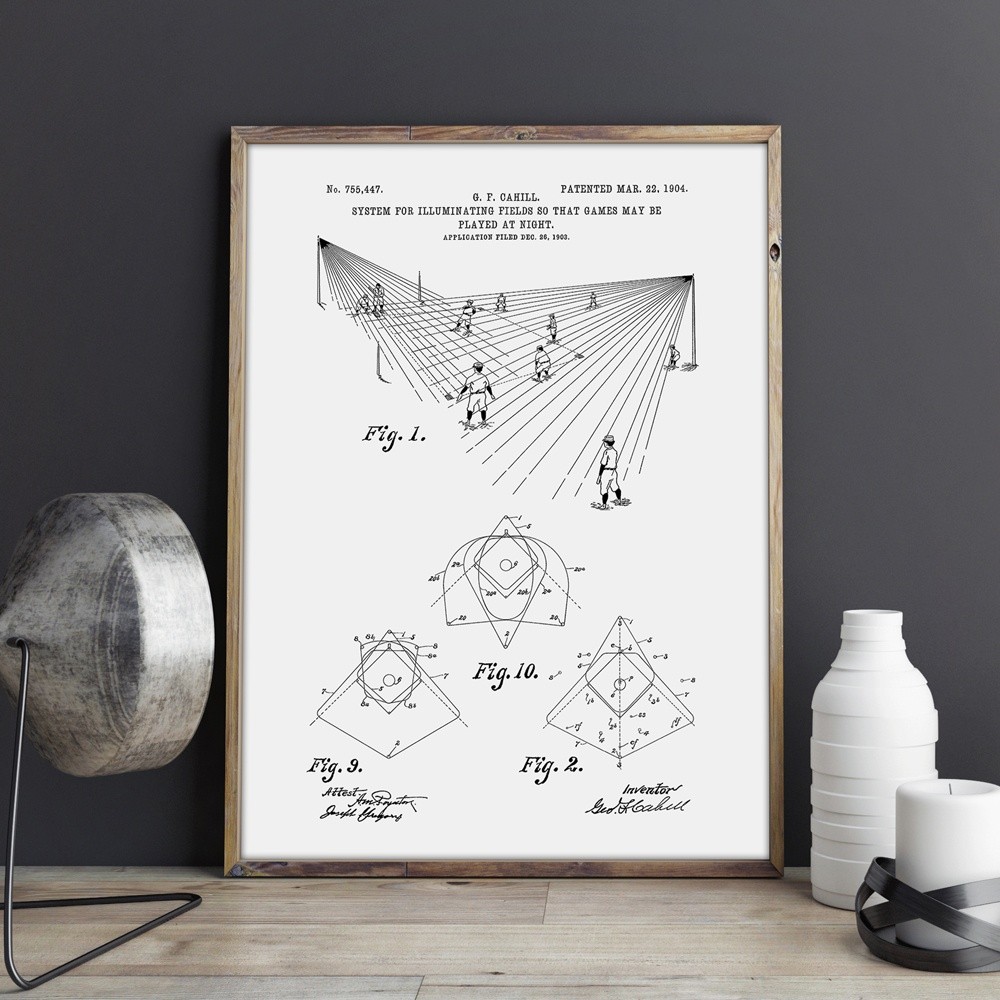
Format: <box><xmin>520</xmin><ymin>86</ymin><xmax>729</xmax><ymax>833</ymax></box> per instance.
<box><xmin>895</xmin><ymin>779</ymin><xmax>1000</xmax><ymax>951</ymax></box>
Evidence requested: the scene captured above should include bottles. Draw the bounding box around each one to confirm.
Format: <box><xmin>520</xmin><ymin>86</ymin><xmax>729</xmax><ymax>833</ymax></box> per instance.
<box><xmin>811</xmin><ymin>607</ymin><xmax>938</xmax><ymax>912</ymax></box>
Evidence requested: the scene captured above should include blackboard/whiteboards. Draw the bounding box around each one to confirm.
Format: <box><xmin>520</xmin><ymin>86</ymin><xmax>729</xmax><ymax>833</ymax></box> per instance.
<box><xmin>224</xmin><ymin>125</ymin><xmax>785</xmax><ymax>880</ymax></box>
